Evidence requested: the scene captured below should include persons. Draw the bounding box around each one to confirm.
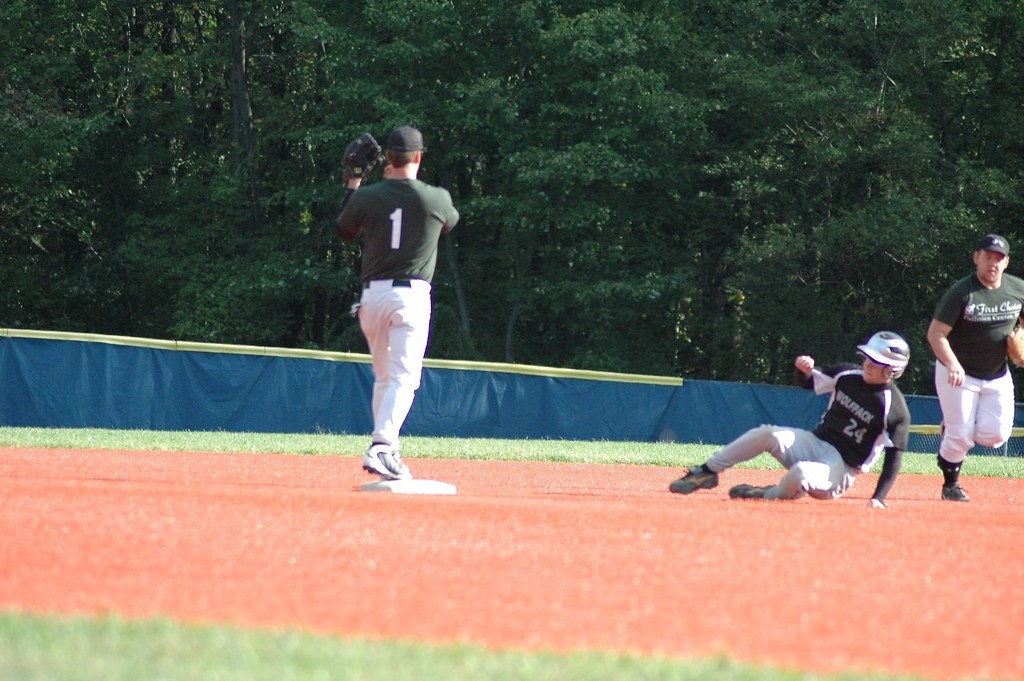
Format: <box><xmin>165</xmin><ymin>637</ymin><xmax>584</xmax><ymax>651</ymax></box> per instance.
<box><xmin>928</xmin><ymin>234</ymin><xmax>1024</xmax><ymax>502</ymax></box>
<box><xmin>334</xmin><ymin>125</ymin><xmax>459</xmax><ymax>480</ymax></box>
<box><xmin>670</xmin><ymin>332</ymin><xmax>910</xmax><ymax>509</ymax></box>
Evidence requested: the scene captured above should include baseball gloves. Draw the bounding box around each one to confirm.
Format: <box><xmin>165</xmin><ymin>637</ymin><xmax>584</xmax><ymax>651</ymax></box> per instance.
<box><xmin>1005</xmin><ymin>325</ymin><xmax>1024</xmax><ymax>371</ymax></box>
<box><xmin>338</xmin><ymin>133</ymin><xmax>385</xmax><ymax>183</ymax></box>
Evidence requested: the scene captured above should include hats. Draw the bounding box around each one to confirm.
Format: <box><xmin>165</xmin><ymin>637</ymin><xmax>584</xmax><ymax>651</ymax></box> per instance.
<box><xmin>974</xmin><ymin>233</ymin><xmax>1009</xmax><ymax>256</ymax></box>
<box><xmin>387</xmin><ymin>126</ymin><xmax>423</xmax><ymax>152</ymax></box>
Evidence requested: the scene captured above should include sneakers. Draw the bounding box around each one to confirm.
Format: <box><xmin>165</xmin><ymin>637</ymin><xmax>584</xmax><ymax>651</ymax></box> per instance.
<box><xmin>941</xmin><ymin>483</ymin><xmax>968</xmax><ymax>502</ymax></box>
<box><xmin>669</xmin><ymin>466</ymin><xmax>718</xmax><ymax>494</ymax></box>
<box><xmin>728</xmin><ymin>484</ymin><xmax>775</xmax><ymax>500</ymax></box>
<box><xmin>363</xmin><ymin>443</ymin><xmax>409</xmax><ymax>480</ymax></box>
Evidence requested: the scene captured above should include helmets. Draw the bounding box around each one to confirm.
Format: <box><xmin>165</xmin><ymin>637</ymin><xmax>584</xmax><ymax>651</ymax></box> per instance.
<box><xmin>856</xmin><ymin>331</ymin><xmax>909</xmax><ymax>379</ymax></box>
